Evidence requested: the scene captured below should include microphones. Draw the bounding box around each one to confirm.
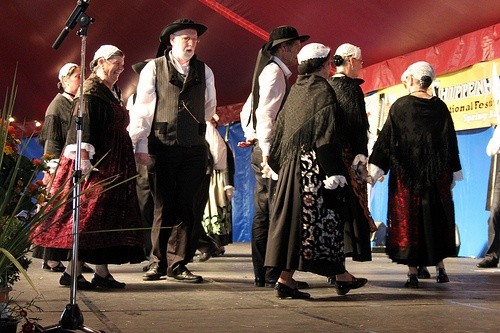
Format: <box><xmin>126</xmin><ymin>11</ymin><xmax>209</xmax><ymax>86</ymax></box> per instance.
<box><xmin>52</xmin><ymin>0</ymin><xmax>90</xmax><ymax>50</ymax></box>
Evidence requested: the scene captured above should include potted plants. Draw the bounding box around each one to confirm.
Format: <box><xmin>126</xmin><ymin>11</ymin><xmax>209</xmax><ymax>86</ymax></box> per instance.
<box><xmin>0</xmin><ymin>216</ymin><xmax>34</xmax><ymax>302</ymax></box>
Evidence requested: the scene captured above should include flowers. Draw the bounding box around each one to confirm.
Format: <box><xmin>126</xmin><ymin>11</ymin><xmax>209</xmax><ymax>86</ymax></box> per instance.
<box><xmin>0</xmin><ymin>60</ymin><xmax>182</xmax><ymax>281</ymax></box>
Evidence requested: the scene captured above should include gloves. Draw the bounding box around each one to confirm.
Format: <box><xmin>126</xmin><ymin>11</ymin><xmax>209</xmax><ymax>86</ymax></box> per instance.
<box><xmin>323</xmin><ymin>175</ymin><xmax>347</xmax><ymax>190</ymax></box>
<box><xmin>259</xmin><ymin>159</ymin><xmax>280</xmax><ymax>182</ymax></box>
<box><xmin>353</xmin><ymin>155</ymin><xmax>379</xmax><ymax>188</ymax></box>
<box><xmin>81</xmin><ymin>159</ymin><xmax>101</xmax><ymax>184</ymax></box>
<box><xmin>45</xmin><ymin>159</ymin><xmax>60</xmax><ymax>175</ymax></box>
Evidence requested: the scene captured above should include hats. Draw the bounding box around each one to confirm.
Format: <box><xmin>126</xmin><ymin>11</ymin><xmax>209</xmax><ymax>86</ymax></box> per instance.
<box><xmin>334</xmin><ymin>42</ymin><xmax>362</xmax><ymax>61</ymax></box>
<box><xmin>92</xmin><ymin>44</ymin><xmax>120</xmax><ymax>60</ymax></box>
<box><xmin>400</xmin><ymin>61</ymin><xmax>435</xmax><ymax>83</ymax></box>
<box><xmin>296</xmin><ymin>42</ymin><xmax>330</xmax><ymax>64</ymax></box>
<box><xmin>158</xmin><ymin>17</ymin><xmax>208</xmax><ymax>49</ymax></box>
<box><xmin>56</xmin><ymin>62</ymin><xmax>78</xmax><ymax>82</ymax></box>
<box><xmin>265</xmin><ymin>24</ymin><xmax>312</xmax><ymax>52</ymax></box>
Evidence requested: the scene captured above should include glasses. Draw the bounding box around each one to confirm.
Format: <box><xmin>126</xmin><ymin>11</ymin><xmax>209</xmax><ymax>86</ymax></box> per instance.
<box><xmin>174</xmin><ymin>35</ymin><xmax>201</xmax><ymax>44</ymax></box>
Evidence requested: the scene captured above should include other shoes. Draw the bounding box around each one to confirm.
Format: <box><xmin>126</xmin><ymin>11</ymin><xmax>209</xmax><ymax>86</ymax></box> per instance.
<box><xmin>165</xmin><ymin>269</ymin><xmax>204</xmax><ymax>283</ymax></box>
<box><xmin>141</xmin><ymin>265</ymin><xmax>162</xmax><ymax>281</ymax></box>
<box><xmin>41</xmin><ymin>259</ymin><xmax>67</xmax><ymax>272</ymax></box>
<box><xmin>60</xmin><ymin>270</ymin><xmax>97</xmax><ymax>290</ymax></box>
<box><xmin>196</xmin><ymin>245</ymin><xmax>217</xmax><ymax>261</ymax></box>
<box><xmin>264</xmin><ymin>277</ymin><xmax>310</xmax><ymax>290</ymax></box>
<box><xmin>82</xmin><ymin>265</ymin><xmax>93</xmax><ymax>273</ymax></box>
<box><xmin>254</xmin><ymin>277</ymin><xmax>264</xmax><ymax>288</ymax></box>
<box><xmin>143</xmin><ymin>265</ymin><xmax>151</xmax><ymax>272</ymax></box>
<box><xmin>474</xmin><ymin>259</ymin><xmax>496</xmax><ymax>269</ymax></box>
<box><xmin>91</xmin><ymin>272</ymin><xmax>126</xmax><ymax>289</ymax></box>
<box><xmin>404</xmin><ymin>266</ymin><xmax>449</xmax><ymax>289</ymax></box>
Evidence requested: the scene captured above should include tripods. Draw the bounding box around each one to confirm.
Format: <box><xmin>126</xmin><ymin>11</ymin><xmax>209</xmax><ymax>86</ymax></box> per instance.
<box><xmin>28</xmin><ymin>38</ymin><xmax>102</xmax><ymax>333</ymax></box>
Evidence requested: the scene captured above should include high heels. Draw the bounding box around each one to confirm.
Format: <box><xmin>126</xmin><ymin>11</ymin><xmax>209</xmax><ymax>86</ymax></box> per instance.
<box><xmin>336</xmin><ymin>277</ymin><xmax>369</xmax><ymax>297</ymax></box>
<box><xmin>274</xmin><ymin>283</ymin><xmax>311</xmax><ymax>300</ymax></box>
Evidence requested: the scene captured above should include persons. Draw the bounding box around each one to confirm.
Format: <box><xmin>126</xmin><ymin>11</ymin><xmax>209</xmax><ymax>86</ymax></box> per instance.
<box><xmin>475</xmin><ymin>121</ymin><xmax>500</xmax><ymax>268</ymax></box>
<box><xmin>124</xmin><ymin>17</ymin><xmax>236</xmax><ymax>282</ymax></box>
<box><xmin>26</xmin><ymin>62</ymin><xmax>94</xmax><ymax>274</ymax></box>
<box><xmin>368</xmin><ymin>61</ymin><xmax>464</xmax><ymax>290</ymax></box>
<box><xmin>327</xmin><ymin>42</ymin><xmax>370</xmax><ymax>287</ymax></box>
<box><xmin>42</xmin><ymin>45</ymin><xmax>150</xmax><ymax>293</ymax></box>
<box><xmin>236</xmin><ymin>24</ymin><xmax>312</xmax><ymax>291</ymax></box>
<box><xmin>261</xmin><ymin>42</ymin><xmax>368</xmax><ymax>299</ymax></box>
